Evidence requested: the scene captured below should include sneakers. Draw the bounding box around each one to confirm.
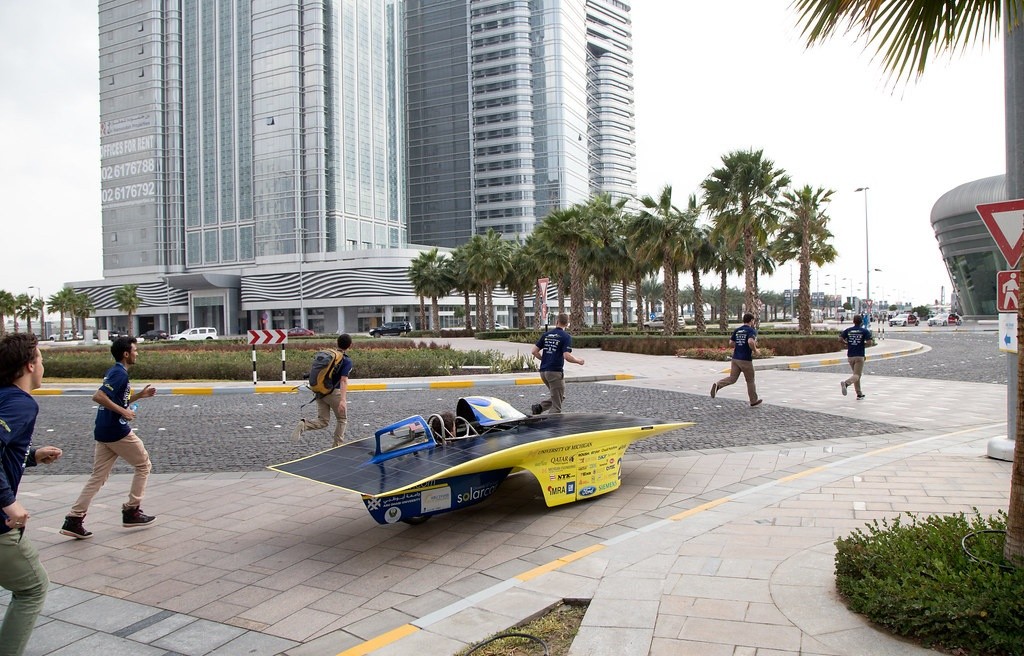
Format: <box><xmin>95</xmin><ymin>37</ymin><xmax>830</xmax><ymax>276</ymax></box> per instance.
<box><xmin>59</xmin><ymin>514</ymin><xmax>93</xmax><ymax>539</ymax></box>
<box><xmin>122</xmin><ymin>505</ymin><xmax>156</xmax><ymax>527</ymax></box>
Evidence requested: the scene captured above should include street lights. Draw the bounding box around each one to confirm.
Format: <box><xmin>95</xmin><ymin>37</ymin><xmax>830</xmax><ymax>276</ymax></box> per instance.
<box><xmin>28</xmin><ymin>286</ymin><xmax>40</xmax><ymax>300</ymax></box>
<box><xmin>855</xmin><ymin>187</ymin><xmax>871</xmax><ymax>327</ymax></box>
<box><xmin>157</xmin><ymin>275</ymin><xmax>171</xmax><ymax>339</ymax></box>
<box><xmin>826</xmin><ymin>274</ymin><xmax>852</xmax><ymax>304</ymax></box>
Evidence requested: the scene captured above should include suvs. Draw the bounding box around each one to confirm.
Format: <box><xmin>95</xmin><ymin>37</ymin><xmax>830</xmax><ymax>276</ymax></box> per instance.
<box><xmin>642</xmin><ymin>314</ymin><xmax>686</xmax><ymax>331</ymax></box>
<box><xmin>167</xmin><ymin>327</ymin><xmax>219</xmax><ymax>341</ymax></box>
<box><xmin>369</xmin><ymin>321</ymin><xmax>412</xmax><ymax>339</ymax></box>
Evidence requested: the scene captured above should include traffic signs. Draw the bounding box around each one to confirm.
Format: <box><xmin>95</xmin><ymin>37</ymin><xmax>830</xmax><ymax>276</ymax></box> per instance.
<box><xmin>999</xmin><ymin>314</ymin><xmax>1018</xmax><ymax>354</ymax></box>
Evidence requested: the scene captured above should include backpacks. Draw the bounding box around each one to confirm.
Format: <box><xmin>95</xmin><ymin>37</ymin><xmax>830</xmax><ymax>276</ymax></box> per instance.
<box><xmin>309</xmin><ymin>350</ymin><xmax>348</xmax><ymax>395</ymax></box>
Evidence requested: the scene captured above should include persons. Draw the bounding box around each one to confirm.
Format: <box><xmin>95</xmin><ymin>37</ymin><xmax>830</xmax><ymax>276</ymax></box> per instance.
<box><xmin>531</xmin><ymin>313</ymin><xmax>584</xmax><ymax>414</ymax></box>
<box><xmin>840</xmin><ymin>311</ymin><xmax>885</xmax><ymax>332</ymax></box>
<box><xmin>58</xmin><ymin>336</ymin><xmax>156</xmax><ymax>539</ymax></box>
<box><xmin>840</xmin><ymin>315</ymin><xmax>873</xmax><ymax>400</ymax></box>
<box><xmin>290</xmin><ymin>334</ymin><xmax>352</xmax><ymax>448</ymax></box>
<box><xmin>710</xmin><ymin>312</ymin><xmax>762</xmax><ymax>407</ymax></box>
<box><xmin>0</xmin><ymin>332</ymin><xmax>63</xmax><ymax>656</ymax></box>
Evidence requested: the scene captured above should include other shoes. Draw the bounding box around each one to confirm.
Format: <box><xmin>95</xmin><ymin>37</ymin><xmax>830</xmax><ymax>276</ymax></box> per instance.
<box><xmin>841</xmin><ymin>381</ymin><xmax>847</xmax><ymax>396</ymax></box>
<box><xmin>711</xmin><ymin>383</ymin><xmax>717</xmax><ymax>398</ymax></box>
<box><xmin>857</xmin><ymin>395</ymin><xmax>865</xmax><ymax>400</ymax></box>
<box><xmin>291</xmin><ymin>421</ymin><xmax>304</xmax><ymax>444</ymax></box>
<box><xmin>750</xmin><ymin>400</ymin><xmax>762</xmax><ymax>406</ymax></box>
<box><xmin>532</xmin><ymin>404</ymin><xmax>542</xmax><ymax>415</ymax></box>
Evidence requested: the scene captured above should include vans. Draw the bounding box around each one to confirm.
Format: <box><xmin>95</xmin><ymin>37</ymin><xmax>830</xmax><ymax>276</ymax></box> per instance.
<box><xmin>810</xmin><ymin>309</ymin><xmax>823</xmax><ymax>323</ymax></box>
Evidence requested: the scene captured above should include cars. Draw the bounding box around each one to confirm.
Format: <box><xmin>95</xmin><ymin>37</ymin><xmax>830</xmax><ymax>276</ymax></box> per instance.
<box><xmin>48</xmin><ymin>329</ymin><xmax>83</xmax><ymax>342</ymax></box>
<box><xmin>287</xmin><ymin>327</ymin><xmax>315</xmax><ymax>337</ymax></box>
<box><xmin>888</xmin><ymin>313</ymin><xmax>921</xmax><ymax>327</ymax></box>
<box><xmin>108</xmin><ymin>330</ymin><xmax>129</xmax><ymax>342</ymax></box>
<box><xmin>927</xmin><ymin>312</ymin><xmax>963</xmax><ymax>326</ymax></box>
<box><xmin>485</xmin><ymin>323</ymin><xmax>509</xmax><ymax>330</ymax></box>
<box><xmin>140</xmin><ymin>330</ymin><xmax>169</xmax><ymax>341</ymax></box>
